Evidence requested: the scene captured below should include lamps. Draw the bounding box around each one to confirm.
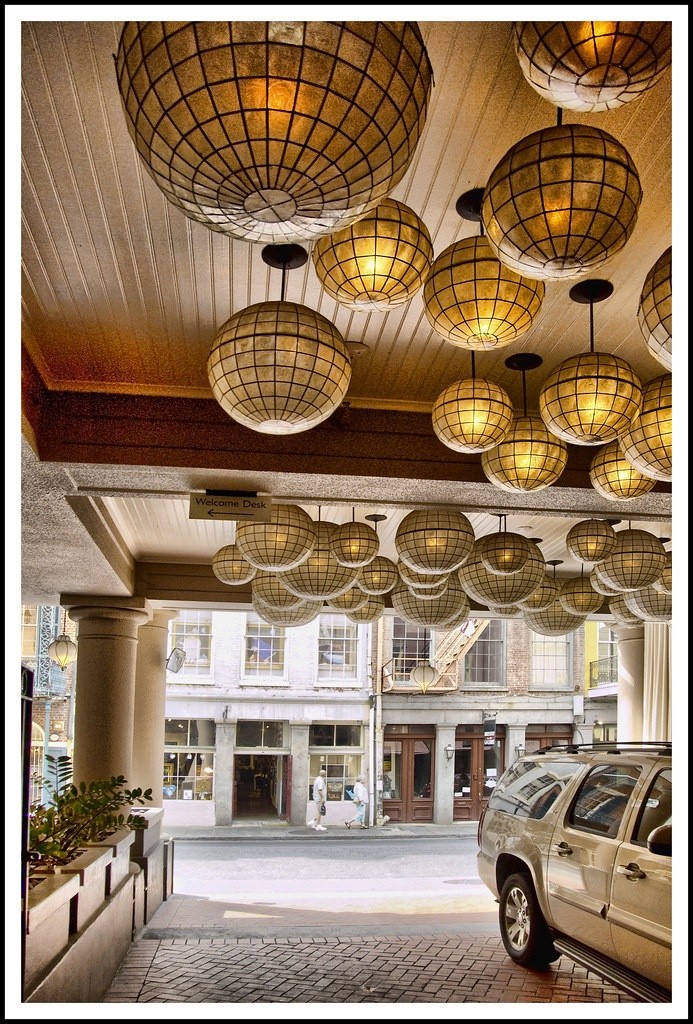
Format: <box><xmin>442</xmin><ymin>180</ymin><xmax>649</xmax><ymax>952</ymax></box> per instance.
<box><xmin>410</xmin><ymin>629</ymin><xmax>440</xmax><ymax>696</ymax></box>
<box><xmin>49</xmin><ymin>607</ymin><xmax>77</xmax><ymax>672</ymax></box>
<box><xmin>113</xmin><ymin>22</ymin><xmax>672</xmax><ymax>499</ymax></box>
<box><xmin>212</xmin><ymin>509</ymin><xmax>672</xmax><ymax>636</ymax></box>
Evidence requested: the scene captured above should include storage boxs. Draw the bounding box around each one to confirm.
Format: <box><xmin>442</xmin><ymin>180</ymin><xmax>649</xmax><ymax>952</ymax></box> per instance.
<box><xmin>462</xmin><ymin>787</ymin><xmax>470</xmax><ymax>792</ymax></box>
<box><xmin>183</xmin><ymin>790</ymin><xmax>192</xmax><ymax>799</ymax></box>
<box><xmin>455</xmin><ymin>793</ymin><xmax>463</xmax><ymax>796</ymax></box>
<box><xmin>486</xmin><ymin>769</ymin><xmax>497</xmax><ymax>776</ymax></box>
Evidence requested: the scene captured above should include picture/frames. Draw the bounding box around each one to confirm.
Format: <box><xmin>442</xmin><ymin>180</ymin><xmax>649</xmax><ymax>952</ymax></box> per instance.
<box><xmin>163</xmin><ymin>763</ymin><xmax>174</xmax><ymax>785</ymax></box>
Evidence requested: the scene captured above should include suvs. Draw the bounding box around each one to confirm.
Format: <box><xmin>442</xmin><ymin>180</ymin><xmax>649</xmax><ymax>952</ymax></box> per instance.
<box><xmin>477</xmin><ymin>740</ymin><xmax>673</xmax><ymax>1003</ymax></box>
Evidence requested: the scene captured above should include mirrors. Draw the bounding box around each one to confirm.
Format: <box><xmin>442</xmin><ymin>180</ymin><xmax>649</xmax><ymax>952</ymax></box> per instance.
<box><xmin>166</xmin><ymin>648</ymin><xmax>186</xmax><ymax>675</ymax></box>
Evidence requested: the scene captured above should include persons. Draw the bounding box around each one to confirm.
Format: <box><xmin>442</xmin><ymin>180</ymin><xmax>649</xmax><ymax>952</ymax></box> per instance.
<box><xmin>309</xmin><ymin>769</ymin><xmax>327</xmax><ymax>831</ymax></box>
<box><xmin>345</xmin><ymin>773</ymin><xmax>369</xmax><ymax>829</ymax></box>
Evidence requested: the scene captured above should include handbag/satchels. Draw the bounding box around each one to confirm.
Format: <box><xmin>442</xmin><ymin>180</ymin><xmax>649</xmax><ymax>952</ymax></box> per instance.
<box><xmin>353</xmin><ymin>799</ymin><xmax>361</xmax><ymax>805</ymax></box>
<box><xmin>321</xmin><ymin>803</ymin><xmax>326</xmax><ymax>816</ymax></box>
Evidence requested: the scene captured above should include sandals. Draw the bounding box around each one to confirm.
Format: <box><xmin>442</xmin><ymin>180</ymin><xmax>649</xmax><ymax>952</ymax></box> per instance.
<box><xmin>344</xmin><ymin>821</ymin><xmax>351</xmax><ymax>829</ymax></box>
<box><xmin>360</xmin><ymin>825</ymin><xmax>370</xmax><ymax>829</ymax></box>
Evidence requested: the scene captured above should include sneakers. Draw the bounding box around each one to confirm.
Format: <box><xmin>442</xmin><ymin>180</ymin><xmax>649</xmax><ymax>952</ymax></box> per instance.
<box><xmin>312</xmin><ymin>824</ymin><xmax>327</xmax><ymax>830</ymax></box>
